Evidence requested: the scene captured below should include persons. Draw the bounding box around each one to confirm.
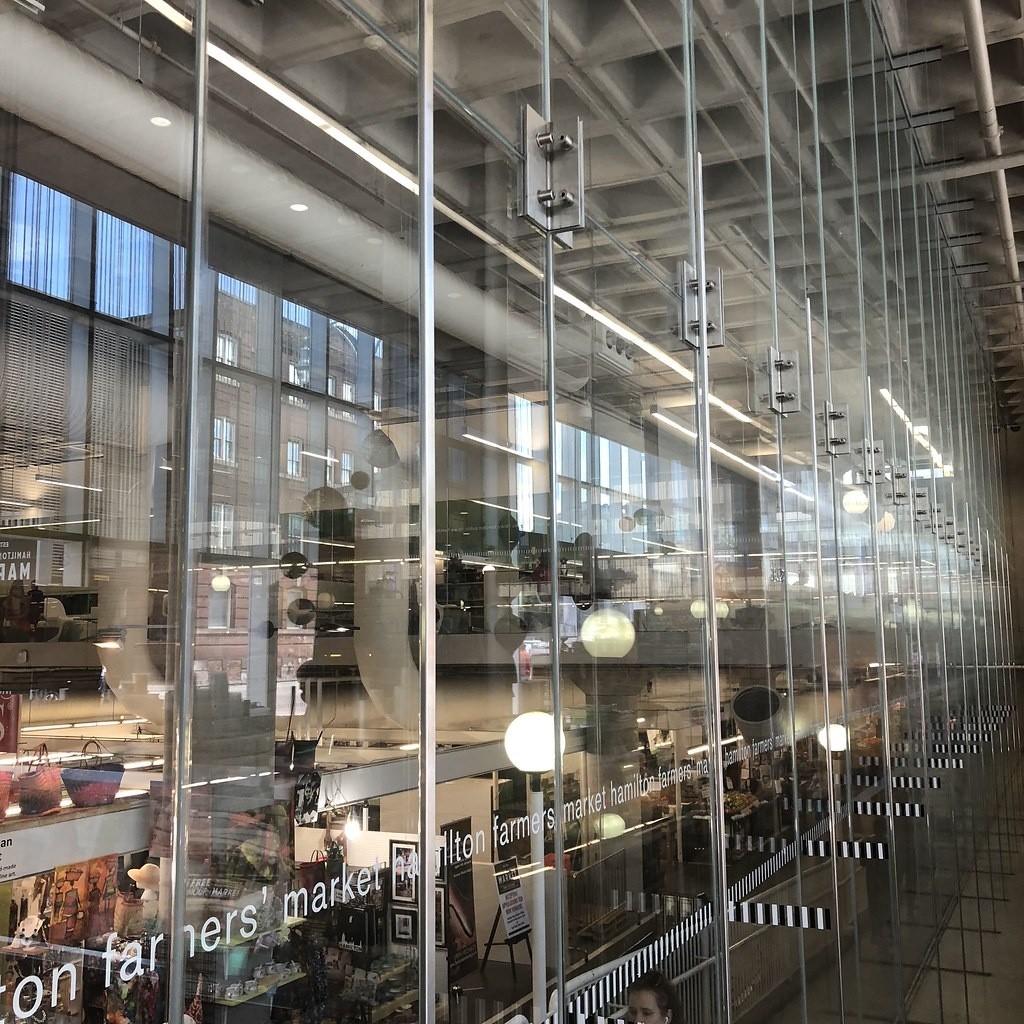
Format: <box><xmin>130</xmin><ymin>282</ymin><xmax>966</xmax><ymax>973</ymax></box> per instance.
<box><xmin>626</xmin><ymin>970</ymin><xmax>684</xmax><ymax>1024</ymax></box>
<box><xmin>726</xmin><ymin>745</ymin><xmax>742</xmax><ymax>790</ymax></box>
<box><xmin>809</xmin><ymin>772</ymin><xmax>830</xmax><ymax>822</ymax></box>
<box><xmin>395</xmin><ymin>849</ymin><xmax>414</xmax><ymax>891</ymax></box>
<box><xmin>126</xmin><ymin>860</ymin><xmax>161</xmax><ymax>919</ymax></box>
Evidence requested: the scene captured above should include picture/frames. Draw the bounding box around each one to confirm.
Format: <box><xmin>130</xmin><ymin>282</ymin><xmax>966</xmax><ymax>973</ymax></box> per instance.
<box><xmin>389</xmin><ymin>839</ymin><xmax>419</xmax><ymax>907</ymax></box>
<box><xmin>390</xmin><ymin>908</ymin><xmax>417</xmax><ymax>946</ymax></box>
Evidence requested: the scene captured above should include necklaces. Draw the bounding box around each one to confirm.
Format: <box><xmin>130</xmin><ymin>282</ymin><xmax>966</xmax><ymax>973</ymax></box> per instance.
<box><xmin>19</xmin><ymin>919</ymin><xmax>41</xmax><ymax>941</ymax></box>
<box><xmin>31</xmin><ymin>1010</ymin><xmax>47</xmax><ymax>1023</ymax></box>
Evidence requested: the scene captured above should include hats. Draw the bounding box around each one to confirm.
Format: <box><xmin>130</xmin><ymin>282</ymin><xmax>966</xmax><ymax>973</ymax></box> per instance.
<box><xmin>127</xmin><ymin>863</ymin><xmax>160</xmax><ymax>892</ymax></box>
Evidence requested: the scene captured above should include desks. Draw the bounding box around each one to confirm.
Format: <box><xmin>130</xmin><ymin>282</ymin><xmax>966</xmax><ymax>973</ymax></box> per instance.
<box><xmin>691</xmin><ymin>794</ymin><xmax>780</xmax><ymax>859</ymax></box>
<box><xmin>208</xmin><ymin>972</ymin><xmax>309</xmax><ymax>1024</ymax></box>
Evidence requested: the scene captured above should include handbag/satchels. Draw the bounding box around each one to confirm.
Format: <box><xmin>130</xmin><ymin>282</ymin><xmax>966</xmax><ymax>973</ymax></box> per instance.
<box><xmin>326</xmin><ymin>841</ymin><xmax>347</xmax><ymax>888</ymax></box>
<box><xmin>299</xmin><ymin>850</ymin><xmax>326</xmax><ymax>895</ymax></box>
<box><xmin>17</xmin><ymin>743</ymin><xmax>62</xmax><ymax>818</ymax></box>
<box><xmin>60</xmin><ymin>740</ymin><xmax>125</xmax><ymax>806</ymax></box>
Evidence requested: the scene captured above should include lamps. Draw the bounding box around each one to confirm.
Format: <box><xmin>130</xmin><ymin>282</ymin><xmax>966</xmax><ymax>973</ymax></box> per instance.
<box><xmin>690</xmin><ymin>597</ymin><xmax>707</xmax><ymax>619</ymax></box>
<box><xmin>34</xmin><ymin>475</ymin><xmax>104</xmax><ymax>493</ymax></box>
<box><xmin>148</xmin><ymin>0</ymin><xmax>193</xmax><ymax>35</ymax></box>
<box><xmin>715</xmin><ymin>601</ymin><xmax>729</xmax><ymax>619</ymax></box>
<box><xmin>579</xmin><ymin>608</ymin><xmax>636</xmax><ymax>658</ymax></box>
<box><xmin>211</xmin><ymin>575</ymin><xmax>230</xmax><ymax>591</ymax></box>
<box><xmin>435</xmin><ymin>199</ymin><xmax>952</xmax><ymax>534</ymax></box>
<box><xmin>818</xmin><ymin>723</ymin><xmax>851</xmax><ymax>758</ymax></box>
<box><xmin>206</xmin><ymin>40</ymin><xmax>421</xmax><ymax>195</ymax></box>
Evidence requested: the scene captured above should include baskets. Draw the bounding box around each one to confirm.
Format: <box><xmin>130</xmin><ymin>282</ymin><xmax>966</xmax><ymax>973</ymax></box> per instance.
<box><xmin>0</xmin><ymin>771</ymin><xmax>19</xmax><ymax>818</ymax></box>
